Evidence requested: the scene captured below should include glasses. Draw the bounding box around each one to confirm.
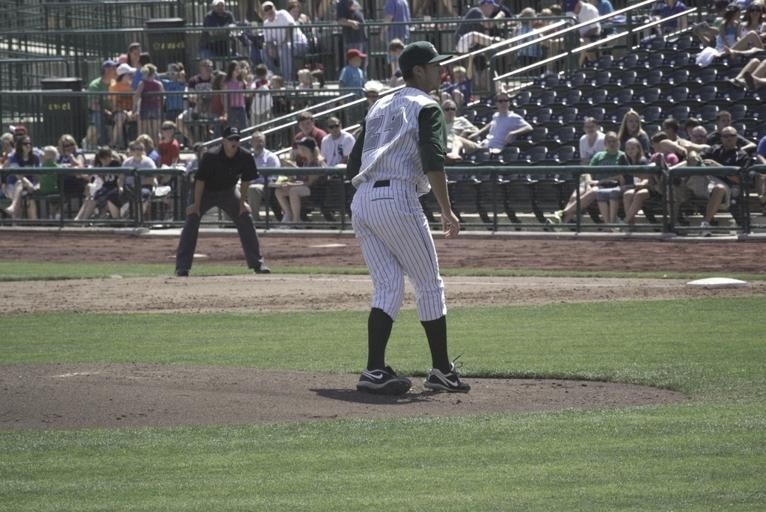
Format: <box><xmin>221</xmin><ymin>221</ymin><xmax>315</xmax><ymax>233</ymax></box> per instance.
<box><xmin>496</xmin><ymin>99</ymin><xmax>509</xmax><ymax>103</ymax></box>
<box><xmin>227</xmin><ymin>136</ymin><xmax>240</xmax><ymax>141</ymax></box>
<box><xmin>327</xmin><ymin>121</ymin><xmax>342</xmax><ymax>128</ymax></box>
<box><xmin>162</xmin><ymin>126</ymin><xmax>172</xmax><ymax>130</ymax></box>
<box><xmin>444</xmin><ymin>107</ymin><xmax>455</xmax><ymax>111</ymax></box>
<box><xmin>64</xmin><ymin>144</ymin><xmax>73</xmax><ymax>147</ymax></box>
<box><xmin>721</xmin><ymin>133</ymin><xmax>737</xmax><ymax>138</ymax></box>
<box><xmin>21</xmin><ymin>142</ymin><xmax>32</xmax><ymax>146</ymax></box>
<box><xmin>129</xmin><ymin>146</ymin><xmax>142</xmax><ymax>152</ymax></box>
<box><xmin>751</xmin><ymin>8</ymin><xmax>761</xmax><ymax>12</ymax></box>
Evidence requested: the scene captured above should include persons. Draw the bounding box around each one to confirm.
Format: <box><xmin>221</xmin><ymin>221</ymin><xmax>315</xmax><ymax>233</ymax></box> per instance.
<box><xmin>341</xmin><ymin>38</ymin><xmax>476</xmax><ymax>395</ymax></box>
<box><xmin>2</xmin><ymin>0</ymin><xmax>765</xmax><ymax>241</ymax></box>
<box><xmin>172</xmin><ymin>124</ymin><xmax>271</xmax><ymax>277</ymax></box>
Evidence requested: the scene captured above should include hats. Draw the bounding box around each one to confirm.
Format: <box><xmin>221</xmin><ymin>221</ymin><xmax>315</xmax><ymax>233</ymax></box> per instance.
<box><xmin>564</xmin><ymin>0</ymin><xmax>579</xmax><ymax>12</ymax></box>
<box><xmin>222</xmin><ymin>126</ymin><xmax>242</xmax><ymax>139</ymax></box>
<box><xmin>262</xmin><ymin>1</ymin><xmax>274</xmax><ymax>12</ymax></box>
<box><xmin>481</xmin><ymin>0</ymin><xmax>499</xmax><ymax>7</ymax></box>
<box><xmin>398</xmin><ymin>40</ymin><xmax>453</xmax><ymax>73</ymax></box>
<box><xmin>15</xmin><ymin>125</ymin><xmax>28</xmax><ymax>132</ymax></box>
<box><xmin>295</xmin><ymin>136</ymin><xmax>317</xmax><ymax>149</ymax></box>
<box><xmin>346</xmin><ymin>48</ymin><xmax>368</xmax><ymax>60</ymax></box>
<box><xmin>101</xmin><ymin>61</ymin><xmax>120</xmax><ymax>70</ymax></box>
<box><xmin>116</xmin><ymin>54</ymin><xmax>137</xmax><ymax>76</ymax></box>
<box><xmin>212</xmin><ymin>0</ymin><xmax>226</xmax><ymax>6</ymax></box>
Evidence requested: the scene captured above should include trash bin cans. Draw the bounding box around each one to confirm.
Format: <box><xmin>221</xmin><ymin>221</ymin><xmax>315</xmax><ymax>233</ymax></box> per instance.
<box><xmin>144</xmin><ymin>17</ymin><xmax>186</xmax><ymax>73</ymax></box>
<box><xmin>39</xmin><ymin>77</ymin><xmax>87</xmax><ymax>145</ymax></box>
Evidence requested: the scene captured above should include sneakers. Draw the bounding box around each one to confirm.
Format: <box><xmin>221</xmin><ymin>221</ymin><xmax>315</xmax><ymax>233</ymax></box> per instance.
<box><xmin>545</xmin><ymin>215</ymin><xmax>564</xmax><ymax>232</ymax></box>
<box><xmin>254</xmin><ymin>265</ymin><xmax>270</xmax><ymax>274</ymax></box>
<box><xmin>700</xmin><ymin>221</ymin><xmax>712</xmax><ymax>237</ymax></box>
<box><xmin>423</xmin><ymin>360</ymin><xmax>470</xmax><ymax>393</ymax></box>
<box><xmin>356</xmin><ymin>366</ymin><xmax>412</xmax><ymax>396</ymax></box>
<box><xmin>176</xmin><ymin>267</ymin><xmax>188</xmax><ymax>276</ymax></box>
